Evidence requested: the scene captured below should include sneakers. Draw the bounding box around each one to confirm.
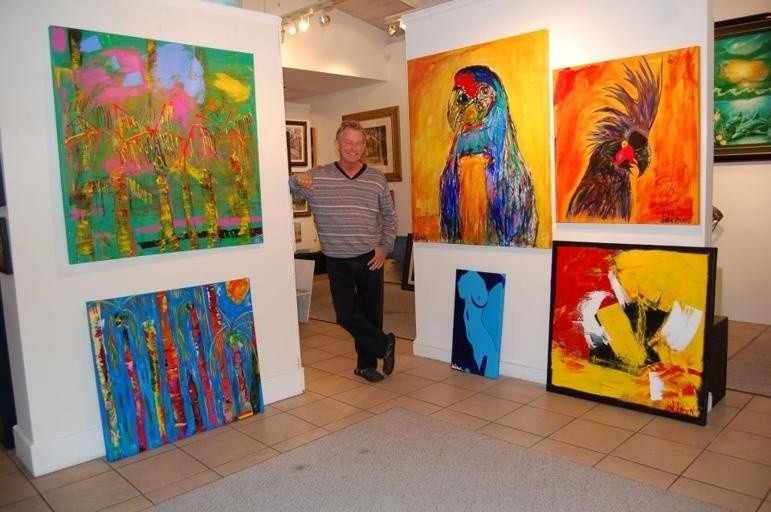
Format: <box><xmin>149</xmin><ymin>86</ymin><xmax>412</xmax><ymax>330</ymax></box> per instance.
<box><xmin>353</xmin><ymin>367</ymin><xmax>384</xmax><ymax>382</ymax></box>
<box><xmin>383</xmin><ymin>333</ymin><xmax>395</xmax><ymax>374</ymax></box>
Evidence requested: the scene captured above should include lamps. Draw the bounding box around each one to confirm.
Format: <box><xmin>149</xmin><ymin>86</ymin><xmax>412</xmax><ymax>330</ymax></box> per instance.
<box><xmin>385</xmin><ymin>22</ymin><xmax>398</xmax><ymax>36</ymax></box>
<box><xmin>280</xmin><ymin>8</ymin><xmax>330</xmax><ymax>43</ymax></box>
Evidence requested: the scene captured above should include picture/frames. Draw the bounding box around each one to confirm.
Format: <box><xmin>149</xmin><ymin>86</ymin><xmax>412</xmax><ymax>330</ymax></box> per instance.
<box><xmin>290</xmin><ymin>172</ymin><xmax>311</xmax><ymax>217</ymax></box>
<box><xmin>714</xmin><ymin>12</ymin><xmax>770</xmax><ymax>165</ymax></box>
<box><xmin>286</xmin><ymin>120</ymin><xmax>307</xmax><ymax>166</ymax></box>
<box><xmin>402</xmin><ymin>233</ymin><xmax>414</xmax><ymax>291</ymax></box>
<box><xmin>546</xmin><ymin>240</ymin><xmax>717</xmax><ymax>426</ymax></box>
<box><xmin>342</xmin><ymin>106</ymin><xmax>402</xmax><ymax>182</ymax></box>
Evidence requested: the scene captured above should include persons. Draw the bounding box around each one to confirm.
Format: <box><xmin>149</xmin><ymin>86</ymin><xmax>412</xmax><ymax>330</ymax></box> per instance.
<box><xmin>289</xmin><ymin>121</ymin><xmax>398</xmax><ymax>383</ymax></box>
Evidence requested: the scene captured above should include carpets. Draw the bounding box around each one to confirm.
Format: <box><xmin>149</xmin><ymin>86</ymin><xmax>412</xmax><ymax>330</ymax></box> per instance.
<box><xmin>309</xmin><ymin>274</ymin><xmax>416</xmax><ymax>339</ymax></box>
<box><xmin>726</xmin><ymin>320</ymin><xmax>771</xmax><ymax>396</ymax></box>
<box><xmin>145</xmin><ymin>406</ymin><xmax>730</xmax><ymax>512</ymax></box>
